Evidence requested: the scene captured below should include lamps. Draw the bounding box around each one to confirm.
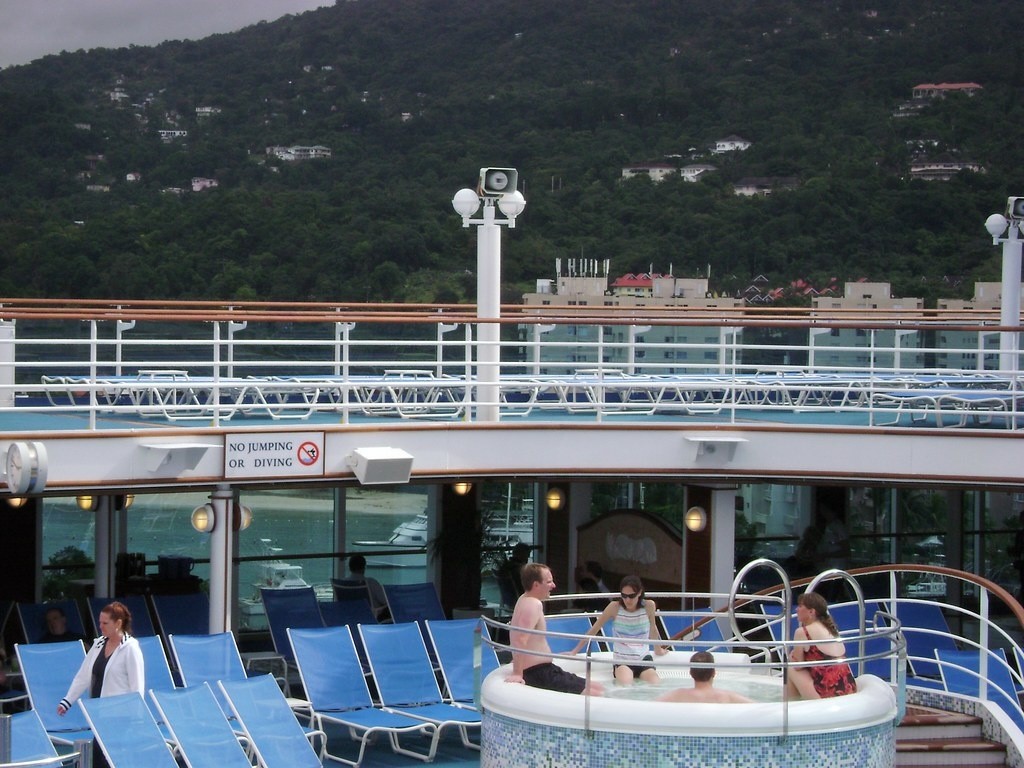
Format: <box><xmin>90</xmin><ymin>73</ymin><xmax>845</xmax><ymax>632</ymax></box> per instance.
<box><xmin>685</xmin><ymin>506</ymin><xmax>708</xmax><ymax>532</ymax></box>
<box><xmin>8</xmin><ymin>497</ymin><xmax>28</xmax><ymax>507</ymax></box>
<box><xmin>76</xmin><ymin>495</ymin><xmax>97</xmax><ymax>511</ymax></box>
<box><xmin>544</xmin><ymin>487</ymin><xmax>566</xmax><ymax>510</ymax></box>
<box><xmin>190</xmin><ymin>503</ymin><xmax>216</xmax><ymax>534</ymax></box>
<box><xmin>454</xmin><ymin>483</ymin><xmax>473</xmax><ymax>496</ymax></box>
<box><xmin>234</xmin><ymin>502</ymin><xmax>252</xmax><ymax>531</ymax></box>
<box><xmin>116</xmin><ymin>494</ymin><xmax>135</xmax><ymax>510</ymax></box>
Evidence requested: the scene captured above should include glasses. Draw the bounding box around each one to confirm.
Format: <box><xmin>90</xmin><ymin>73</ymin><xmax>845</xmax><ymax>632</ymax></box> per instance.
<box><xmin>621</xmin><ymin>591</ymin><xmax>639</xmax><ymax>599</ymax></box>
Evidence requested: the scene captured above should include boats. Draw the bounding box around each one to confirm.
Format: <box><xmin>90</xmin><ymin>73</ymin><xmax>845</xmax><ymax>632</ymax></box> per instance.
<box><xmin>238</xmin><ymin>563</ymin><xmax>335</xmax><ymax>632</ymax></box>
<box><xmin>352</xmin><ymin>497</ymin><xmax>534</xmax><ymax>567</ymax></box>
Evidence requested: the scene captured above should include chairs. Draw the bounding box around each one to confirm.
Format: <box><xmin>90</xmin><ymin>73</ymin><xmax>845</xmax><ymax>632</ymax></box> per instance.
<box><xmin>0</xmin><ymin>575</ymin><xmax>500</xmax><ymax>768</ymax></box>
<box><xmin>594</xmin><ymin>608</ymin><xmax>662</xmax><ymax>652</ymax></box>
<box><xmin>544</xmin><ymin>612</ymin><xmax>601</xmax><ymax>653</ymax></box>
<box><xmin>761</xmin><ymin>601</ymin><xmax>1024</xmax><ymax>733</ymax></box>
<box><xmin>658</xmin><ymin>608</ymin><xmax>728</xmax><ymax>651</ymax></box>
<box><xmin>40</xmin><ymin>372</ymin><xmax>1024</xmax><ymax>427</ymax></box>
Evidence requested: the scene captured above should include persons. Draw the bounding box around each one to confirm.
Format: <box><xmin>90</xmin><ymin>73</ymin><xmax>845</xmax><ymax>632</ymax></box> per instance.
<box><xmin>342</xmin><ymin>554</ymin><xmax>393</xmax><ymax>623</ymax></box>
<box><xmin>560</xmin><ymin>575</ymin><xmax>669</xmax><ymax>684</ymax></box>
<box><xmin>498</xmin><ymin>542</ymin><xmax>613</xmax><ymax>613</ymax></box>
<box><xmin>504</xmin><ymin>563</ymin><xmax>606</xmax><ymax>696</ymax></box>
<box><xmin>38</xmin><ymin>607</ymin><xmax>91</xmax><ymax>653</ymax></box>
<box><xmin>788</xmin><ymin>593</ymin><xmax>856</xmax><ymax>699</ymax></box>
<box><xmin>57</xmin><ymin>601</ymin><xmax>145</xmax><ymax>768</ymax></box>
<box><xmin>656</xmin><ymin>651</ymin><xmax>756</xmax><ymax>702</ymax></box>
<box><xmin>812</xmin><ymin>502</ymin><xmax>855</xmax><ymax>603</ymax></box>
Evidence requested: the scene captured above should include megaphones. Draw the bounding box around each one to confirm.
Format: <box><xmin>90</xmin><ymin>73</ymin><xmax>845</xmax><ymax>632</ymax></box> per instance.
<box><xmin>479</xmin><ymin>167</ymin><xmax>517</xmax><ymax>194</ymax></box>
<box><xmin>1008</xmin><ymin>197</ymin><xmax>1024</xmax><ymax>219</ymax></box>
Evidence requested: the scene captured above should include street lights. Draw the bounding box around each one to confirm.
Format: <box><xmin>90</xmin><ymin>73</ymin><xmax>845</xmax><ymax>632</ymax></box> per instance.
<box><xmin>984</xmin><ymin>197</ymin><xmax>1023</xmax><ymax>372</ymax></box>
<box><xmin>451</xmin><ymin>166</ymin><xmax>528</xmax><ymax>419</ymax></box>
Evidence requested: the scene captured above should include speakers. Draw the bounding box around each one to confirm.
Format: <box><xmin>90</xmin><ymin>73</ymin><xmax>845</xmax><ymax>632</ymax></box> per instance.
<box><xmin>351</xmin><ymin>447</ymin><xmax>414</xmax><ymax>485</ymax></box>
